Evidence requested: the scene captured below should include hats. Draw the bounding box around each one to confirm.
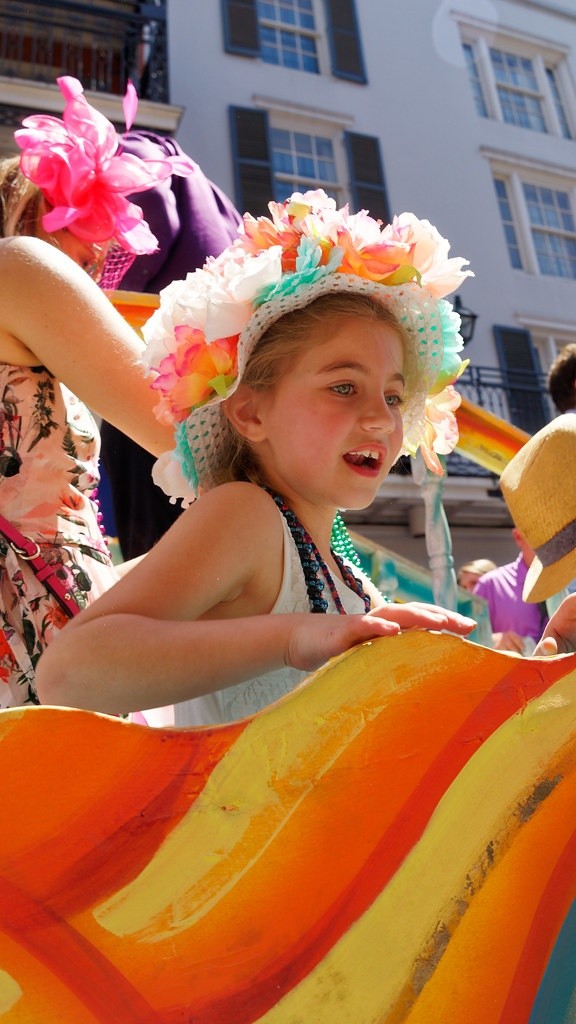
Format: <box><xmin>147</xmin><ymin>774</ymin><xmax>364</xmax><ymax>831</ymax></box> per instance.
<box><xmin>136</xmin><ymin>188</ymin><xmax>475</xmax><ymax>518</ymax></box>
<box><xmin>499</xmin><ymin>411</ymin><xmax>576</xmax><ymax>605</ymax></box>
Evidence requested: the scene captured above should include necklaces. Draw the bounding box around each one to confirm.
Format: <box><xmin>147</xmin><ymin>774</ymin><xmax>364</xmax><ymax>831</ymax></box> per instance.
<box><xmin>329</xmin><ymin>511</ymin><xmax>393</xmax><ymax>604</ymax></box>
<box><xmin>275</xmin><ymin>492</ymin><xmax>371</xmax><ymax>619</ymax></box>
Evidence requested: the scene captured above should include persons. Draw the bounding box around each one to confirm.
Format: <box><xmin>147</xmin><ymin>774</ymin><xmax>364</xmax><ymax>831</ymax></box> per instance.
<box><xmin>34</xmin><ymin>185</ymin><xmax>475</xmax><ymax>727</ymax></box>
<box><xmin>457</xmin><ymin>343</ymin><xmax>576</xmax><ymax>657</ymax></box>
<box><xmin>1</xmin><ymin>74</ymin><xmax>183</xmax><ymax>725</ymax></box>
